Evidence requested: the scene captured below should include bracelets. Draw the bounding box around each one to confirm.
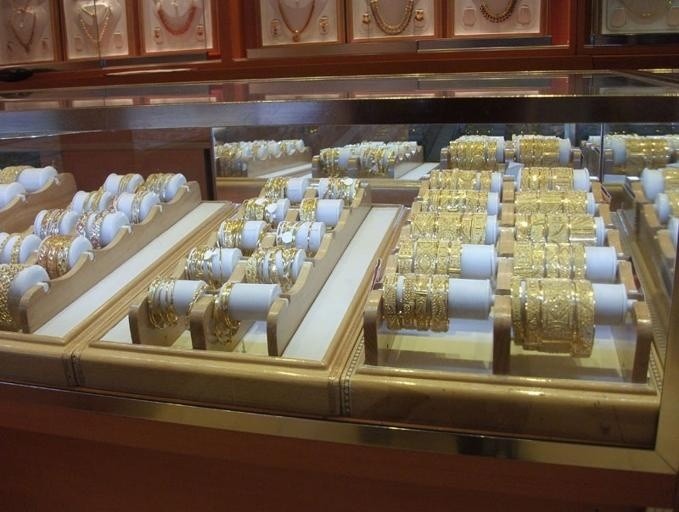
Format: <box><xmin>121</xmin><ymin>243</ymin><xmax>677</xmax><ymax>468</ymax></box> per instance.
<box><xmin>663</xmin><ymin>190</ymin><xmax>679</xmax><ymax>218</ymax></box>
<box><xmin>40</xmin><ymin>208</ymin><xmax>72</xmax><ymax>240</ymax></box>
<box><xmin>460</xmin><ymin>212</ymin><xmax>473</xmax><ymax>243</ymax></box>
<box><xmin>519</xmin><ymin>138</ymin><xmax>560</xmax><ymax>167</ymax></box>
<box><xmin>513</xmin><ymin>240</ymin><xmax>533</xmax><ymax>277</ymax></box>
<box><xmin>37</xmin><ymin>234</ymin><xmax>78</xmax><ymax>280</ymax></box>
<box><xmin>436</xmin><ymin>211</ymin><xmax>463</xmax><ymax>241</ymax></box>
<box><xmin>435</xmin><ymin>239</ymin><xmax>450</xmax><ymax>274</ymax></box>
<box><xmin>510</xmin><ymin>275</ymin><xmax>524</xmax><ymax>347</ymax></box>
<box><xmin>107</xmin><ymin>191</ymin><xmax>128</xmax><ymax>214</ymax></box>
<box><xmin>429</xmin><ymin>274</ymin><xmax>449</xmax><ymax>333</ymax></box>
<box><xmin>327</xmin><ymin>177</ymin><xmax>362</xmax><ymax>206</ymax></box>
<box><xmin>277</xmin><ymin>221</ymin><xmax>315</xmax><ymax>257</ymax></box>
<box><xmin>301</xmin><ymin>198</ymin><xmax>319</xmax><ymax>222</ymax></box>
<box><xmin>398</xmin><ymin>238</ymin><xmax>415</xmax><ymax>274</ymax></box>
<box><xmin>147</xmin><ymin>277</ymin><xmax>180</xmax><ymax>331</ymax></box>
<box><xmin>429</xmin><ymin>169</ymin><xmax>476</xmax><ymax>190</ymax></box>
<box><xmin>0</xmin><ymin>264</ymin><xmax>33</xmax><ymax>332</ymax></box>
<box><xmin>256</xmin><ymin>222</ymin><xmax>271</xmax><ymax>248</ymax></box>
<box><xmin>246</xmin><ymin>245</ymin><xmax>298</xmax><ymax>293</ymax></box>
<box><xmin>362</xmin><ymin>147</ymin><xmax>395</xmax><ymax>177</ymax></box>
<box><xmin>450</xmin><ymin>140</ymin><xmax>457</xmax><ymax>168</ymax></box>
<box><xmin>87</xmin><ymin>210</ymin><xmax>111</xmax><ymax>249</ymax></box>
<box><xmin>480</xmin><ymin>169</ymin><xmax>492</xmax><ymax>192</ymax></box>
<box><xmin>216</xmin><ymin>144</ymin><xmax>241</xmax><ymax>171</ymax></box>
<box><xmin>487</xmin><ymin>140</ymin><xmax>497</xmax><ymax>172</ymax></box>
<box><xmin>74</xmin><ymin>210</ymin><xmax>95</xmax><ymax>237</ymax></box>
<box><xmin>523</xmin><ymin>277</ymin><xmax>541</xmax><ymax>351</ymax></box>
<box><xmin>530</xmin><ymin>167</ymin><xmax>539</xmax><ymax>192</ymax></box>
<box><xmin>0</xmin><ymin>232</ymin><xmax>32</xmax><ymax>264</ymax></box>
<box><xmin>81</xmin><ymin>190</ymin><xmax>106</xmax><ymax>214</ymax></box>
<box><xmin>252</xmin><ymin>142</ymin><xmax>260</xmax><ymax>160</ymax></box>
<box><xmin>264</xmin><ymin>175</ymin><xmax>291</xmax><ymax>199</ymax></box>
<box><xmin>278</xmin><ymin>140</ymin><xmax>289</xmax><ymax>152</ymax></box>
<box><xmin>574</xmin><ymin>278</ymin><xmax>595</xmax><ymax>359</ymax></box>
<box><xmin>466</xmin><ymin>141</ymin><xmax>472</xmax><ymax>171</ymax></box>
<box><xmin>660</xmin><ymin>167</ymin><xmax>679</xmax><ymax>192</ymax></box>
<box><xmin>210</xmin><ymin>282</ymin><xmax>241</xmax><ymax>346</ymax></box>
<box><xmin>563</xmin><ymin>190</ymin><xmax>587</xmax><ymax>215</ymax></box>
<box><xmin>0</xmin><ymin>165</ymin><xmax>33</xmax><ymax>184</ymax></box>
<box><xmin>136</xmin><ymin>172</ymin><xmax>175</xmax><ymax>202</ymax></box>
<box><xmin>448</xmin><ymin>240</ymin><xmax>462</xmax><ymax>278</ymax></box>
<box><xmin>409</xmin><ymin>211</ymin><xmax>437</xmax><ymax>241</ymax></box>
<box><xmin>545</xmin><ymin>242</ymin><xmax>558</xmax><ymax>278</ymax></box>
<box><xmin>558</xmin><ymin>243</ymin><xmax>571</xmax><ymax>279</ymax></box>
<box><xmin>237</xmin><ymin>198</ymin><xmax>279</xmax><ymax>229</ymax></box>
<box><xmin>471</xmin><ymin>212</ymin><xmax>488</xmax><ymax>244</ymax></box>
<box><xmin>542</xmin><ymin>277</ymin><xmax>558</xmax><ymax>353</ymax></box>
<box><xmin>184</xmin><ymin>283</ymin><xmax>208</xmax><ymax>330</ymax></box>
<box><xmin>400</xmin><ymin>273</ymin><xmax>415</xmax><ymax>330</ymax></box>
<box><xmin>129</xmin><ymin>191</ymin><xmax>151</xmax><ymax>224</ymax></box>
<box><xmin>539</xmin><ymin>167</ymin><xmax>574</xmax><ymax>191</ymax></box>
<box><xmin>413</xmin><ymin>239</ymin><xmax>438</xmax><ymax>274</ymax></box>
<box><xmin>533</xmin><ymin>242</ymin><xmax>545</xmax><ymax>278</ymax></box>
<box><xmin>321</xmin><ymin>146</ymin><xmax>340</xmax><ymax>178</ymax></box>
<box><xmin>458</xmin><ymin>140</ymin><xmax>465</xmax><ymax>170</ymax></box>
<box><xmin>382</xmin><ymin>273</ymin><xmax>401</xmax><ymax>330</ymax></box>
<box><xmin>522</xmin><ymin>167</ymin><xmax>529</xmax><ymax>192</ymax></box>
<box><xmin>569</xmin><ymin>213</ymin><xmax>597</xmax><ymax>247</ymax></box>
<box><xmin>184</xmin><ymin>243</ymin><xmax>223</xmax><ymax>288</ymax></box>
<box><xmin>515</xmin><ymin>191</ymin><xmax>539</xmax><ymax>213</ymax></box>
<box><xmin>473</xmin><ymin>141</ymin><xmax>485</xmax><ymax>171</ymax></box>
<box><xmin>117</xmin><ymin>173</ymin><xmax>134</xmax><ymax>198</ymax></box>
<box><xmin>415</xmin><ymin>273</ymin><xmax>432</xmax><ymax>330</ymax></box>
<box><xmin>557</xmin><ymin>281</ymin><xmax>575</xmax><ymax>354</ymax></box>
<box><xmin>216</xmin><ymin>218</ymin><xmax>247</xmax><ymax>249</ymax></box>
<box><xmin>571</xmin><ymin>243</ymin><xmax>587</xmax><ymax>280</ymax></box>
<box><xmin>515</xmin><ymin>212</ymin><xmax>530</xmax><ymax>240</ymax></box>
<box><xmin>619</xmin><ymin>137</ymin><xmax>669</xmax><ymax>176</ymax></box>
<box><xmin>546</xmin><ymin>212</ymin><xmax>570</xmax><ymax>243</ymax></box>
<box><xmin>538</xmin><ymin>191</ymin><xmax>564</xmax><ymax>215</ymax></box>
<box><xmin>530</xmin><ymin>211</ymin><xmax>546</xmax><ymax>243</ymax></box>
<box><xmin>422</xmin><ymin>188</ymin><xmax>489</xmax><ymax>212</ymax></box>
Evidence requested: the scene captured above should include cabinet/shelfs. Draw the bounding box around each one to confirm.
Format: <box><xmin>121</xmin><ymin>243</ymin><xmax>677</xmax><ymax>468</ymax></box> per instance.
<box><xmin>0</xmin><ymin>95</ymin><xmax>679</xmax><ymax>512</ymax></box>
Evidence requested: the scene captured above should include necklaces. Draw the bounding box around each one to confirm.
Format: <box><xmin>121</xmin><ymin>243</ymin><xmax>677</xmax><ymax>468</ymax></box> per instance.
<box><xmin>9</xmin><ymin>7</ymin><xmax>36</xmax><ymax>54</ymax></box>
<box><xmin>79</xmin><ymin>4</ymin><xmax>111</xmax><ymax>44</ymax></box>
<box><xmin>157</xmin><ymin>5</ymin><xmax>196</xmax><ymax>36</ymax></box>
<box><xmin>368</xmin><ymin>0</ymin><xmax>415</xmax><ymax>35</ymax></box>
<box><xmin>479</xmin><ymin>0</ymin><xmax>517</xmax><ymax>24</ymax></box>
<box><xmin>619</xmin><ymin>0</ymin><xmax>674</xmax><ymax>19</ymax></box>
<box><xmin>277</xmin><ymin>0</ymin><xmax>316</xmax><ymax>42</ymax></box>
